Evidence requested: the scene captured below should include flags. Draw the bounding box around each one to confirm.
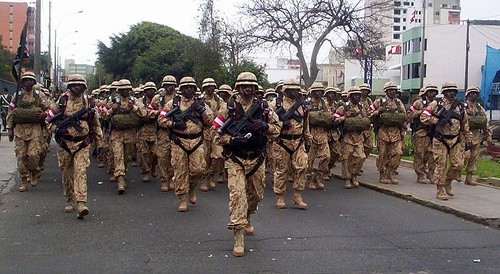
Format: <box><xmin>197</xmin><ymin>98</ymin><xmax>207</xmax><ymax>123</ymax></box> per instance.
<box><xmin>12</xmin><ymin>9</ymin><xmax>31</xmax><ymax>83</ymax></box>
<box><xmin>387</xmin><ymin>45</ymin><xmax>401</xmax><ymax>55</ymax></box>
<box><xmin>410</xmin><ymin>10</ymin><xmax>416</xmax><ymax>24</ymax></box>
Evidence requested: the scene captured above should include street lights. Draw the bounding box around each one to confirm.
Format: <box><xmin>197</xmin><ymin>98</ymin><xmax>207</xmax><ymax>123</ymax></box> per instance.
<box><xmin>51</xmin><ymin>10</ymin><xmax>83</xmax><ymax>90</ymax></box>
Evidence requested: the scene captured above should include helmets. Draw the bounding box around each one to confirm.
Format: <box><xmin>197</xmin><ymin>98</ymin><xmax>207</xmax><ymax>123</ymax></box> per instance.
<box><xmin>66</xmin><ymin>72</ymin><xmax>438</xmax><ymax>98</ymax></box>
<box><xmin>441</xmin><ymin>81</ymin><xmax>458</xmax><ymax>96</ymax></box>
<box><xmin>20</xmin><ymin>71</ymin><xmax>50</xmax><ymax>95</ymax></box>
<box><xmin>464</xmin><ymin>86</ymin><xmax>480</xmax><ymax>96</ymax></box>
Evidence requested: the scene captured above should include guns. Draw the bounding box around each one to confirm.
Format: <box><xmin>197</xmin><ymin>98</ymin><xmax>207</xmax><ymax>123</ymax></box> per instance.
<box><xmin>435</xmin><ymin>97</ymin><xmax>463</xmax><ymax>126</ymax></box>
<box><xmin>217</xmin><ymin>105</ymin><xmax>263</xmax><ymax>146</ymax></box>
<box><xmin>163</xmin><ymin>95</ymin><xmax>206</xmax><ymax>130</ymax></box>
<box><xmin>275</xmin><ymin>100</ymin><xmax>303</xmax><ymax>126</ymax></box>
<box><xmin>48</xmin><ymin>107</ymin><xmax>91</xmax><ymax>133</ymax></box>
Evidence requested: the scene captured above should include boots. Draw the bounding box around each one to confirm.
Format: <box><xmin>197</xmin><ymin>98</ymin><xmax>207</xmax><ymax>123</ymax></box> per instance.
<box><xmin>131</xmin><ymin>161</ymin><xmax>174</xmax><ymax>191</ymax></box>
<box><xmin>417</xmin><ymin>170</ymin><xmax>477</xmax><ymax>200</ymax></box>
<box><xmin>380</xmin><ymin>168</ymin><xmax>399</xmax><ymax>184</ymax></box>
<box><xmin>19</xmin><ymin>166</ymin><xmax>44</xmax><ymax>192</ymax></box>
<box><xmin>64</xmin><ymin>204</ymin><xmax>89</xmax><ymax>219</ymax></box>
<box><xmin>227</xmin><ymin>218</ymin><xmax>254</xmax><ymax>257</ymax></box>
<box><xmin>306</xmin><ymin>174</ymin><xmax>324</xmax><ymax>189</ymax></box>
<box><xmin>178</xmin><ymin>175</ymin><xmax>228</xmax><ymax>211</ymax></box>
<box><xmin>276</xmin><ymin>191</ymin><xmax>307</xmax><ymax>208</ymax></box>
<box><xmin>97</xmin><ymin>162</ymin><xmax>126</xmax><ymax>194</ymax></box>
<box><xmin>344</xmin><ymin>169</ymin><xmax>363</xmax><ymax>188</ymax></box>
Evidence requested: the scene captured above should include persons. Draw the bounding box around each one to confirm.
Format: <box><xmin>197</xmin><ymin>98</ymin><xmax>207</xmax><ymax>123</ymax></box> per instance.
<box><xmin>270</xmin><ymin>78</ymin><xmax>313</xmax><ymax>210</ymax></box>
<box><xmin>419</xmin><ymin>80</ymin><xmax>473</xmax><ymax>200</ymax></box>
<box><xmin>157</xmin><ymin>76</ymin><xmax>214</xmax><ymax>212</ymax></box>
<box><xmin>332</xmin><ymin>85</ymin><xmax>371</xmax><ymax>188</ymax></box>
<box><xmin>0</xmin><ymin>71</ymin><xmax>492</xmax><ymax>257</ymax></box>
<box><xmin>44</xmin><ymin>74</ymin><xmax>104</xmax><ymax>220</ymax></box>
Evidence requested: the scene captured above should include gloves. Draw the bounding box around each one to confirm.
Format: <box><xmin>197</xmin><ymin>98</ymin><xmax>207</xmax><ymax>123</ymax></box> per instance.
<box><xmin>191</xmin><ymin>101</ymin><xmax>206</xmax><ymax>114</ymax></box>
<box><xmin>230</xmin><ymin>135</ymin><xmax>249</xmax><ymax>146</ymax></box>
<box><xmin>173</xmin><ymin>119</ymin><xmax>186</xmax><ymax>131</ymax></box>
<box><xmin>251</xmin><ymin>118</ymin><xmax>269</xmax><ymax>132</ymax></box>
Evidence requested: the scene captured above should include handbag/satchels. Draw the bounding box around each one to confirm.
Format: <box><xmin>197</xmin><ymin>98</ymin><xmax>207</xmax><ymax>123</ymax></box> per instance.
<box><xmin>380</xmin><ymin>112</ymin><xmax>406</xmax><ymax>127</ymax></box>
<box><xmin>111</xmin><ymin>113</ymin><xmax>141</xmax><ymax>129</ymax></box>
<box><xmin>13</xmin><ymin>108</ymin><xmax>45</xmax><ymax>125</ymax></box>
<box><xmin>468</xmin><ymin>115</ymin><xmax>487</xmax><ymax>129</ymax></box>
<box><xmin>342</xmin><ymin>118</ymin><xmax>370</xmax><ymax>132</ymax></box>
<box><xmin>310</xmin><ymin>111</ymin><xmax>334</xmax><ymax>128</ymax></box>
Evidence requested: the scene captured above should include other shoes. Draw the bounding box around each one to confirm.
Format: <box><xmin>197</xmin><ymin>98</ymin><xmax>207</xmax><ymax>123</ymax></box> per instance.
<box><xmin>323</xmin><ymin>173</ymin><xmax>329</xmax><ymax>180</ymax></box>
